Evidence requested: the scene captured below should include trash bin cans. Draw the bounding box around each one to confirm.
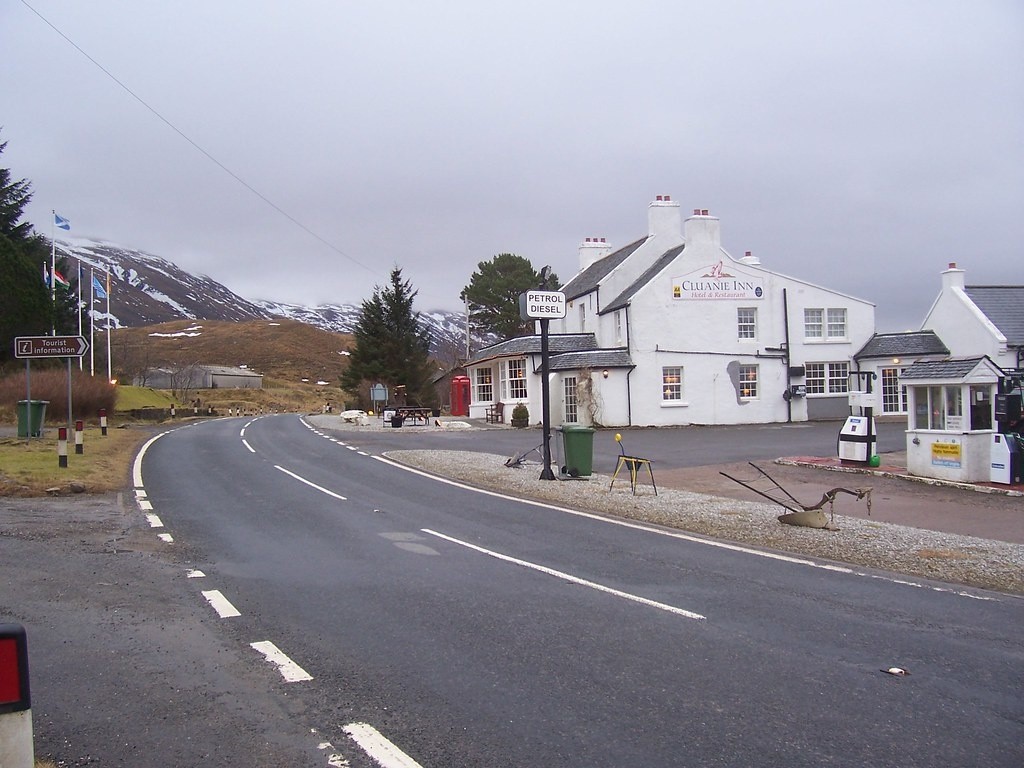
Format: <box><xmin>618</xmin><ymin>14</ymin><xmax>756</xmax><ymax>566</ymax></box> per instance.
<box><xmin>39</xmin><ymin>401</ymin><xmax>50</xmax><ymax>438</ymax></box>
<box><xmin>561</xmin><ymin>426</ymin><xmax>597</xmax><ymax>477</ymax></box>
<box><xmin>17</xmin><ymin>400</ymin><xmax>42</xmax><ymax>438</ymax></box>
<box><xmin>556</xmin><ymin>428</ymin><xmax>566</xmax><ymax>477</ymax></box>
<box><xmin>391</xmin><ymin>416</ymin><xmax>402</xmax><ymax>428</ymax></box>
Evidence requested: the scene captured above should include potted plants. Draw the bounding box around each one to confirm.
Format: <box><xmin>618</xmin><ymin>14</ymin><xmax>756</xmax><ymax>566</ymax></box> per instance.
<box><xmin>511</xmin><ymin>403</ymin><xmax>528</xmax><ymax>426</ymax></box>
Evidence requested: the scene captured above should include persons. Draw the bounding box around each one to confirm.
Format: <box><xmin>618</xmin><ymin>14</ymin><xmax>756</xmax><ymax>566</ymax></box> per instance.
<box><xmin>942</xmin><ymin>399</ymin><xmax>955</xmax><ymax>429</ymax></box>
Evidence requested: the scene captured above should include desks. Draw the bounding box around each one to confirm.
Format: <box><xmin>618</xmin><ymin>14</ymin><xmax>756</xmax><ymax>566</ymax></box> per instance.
<box><xmin>397</xmin><ymin>408</ymin><xmax>432</xmax><ymax>426</ymax></box>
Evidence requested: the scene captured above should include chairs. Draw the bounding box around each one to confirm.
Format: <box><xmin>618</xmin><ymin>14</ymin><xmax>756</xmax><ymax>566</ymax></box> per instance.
<box><xmin>486</xmin><ymin>402</ymin><xmax>505</xmax><ymax>424</ymax></box>
